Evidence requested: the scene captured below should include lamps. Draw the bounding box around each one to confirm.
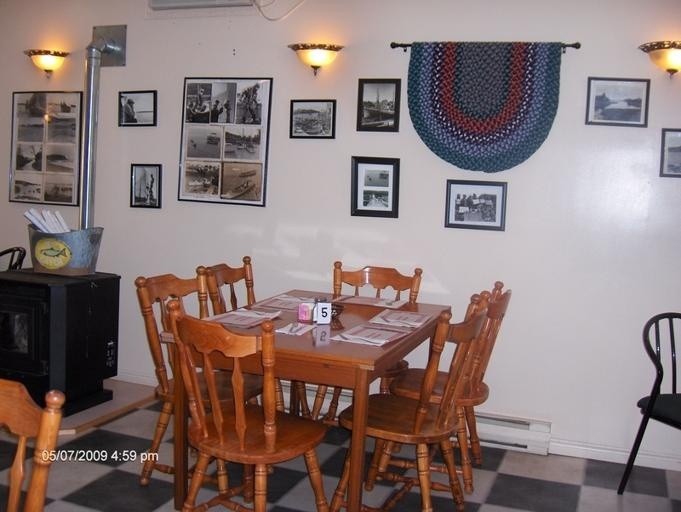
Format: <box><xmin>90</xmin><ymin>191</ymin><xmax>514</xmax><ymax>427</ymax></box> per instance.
<box><xmin>639</xmin><ymin>39</ymin><xmax>681</xmax><ymax>79</ymax></box>
<box><xmin>24</xmin><ymin>47</ymin><xmax>69</xmax><ymax>78</ymax></box>
<box><xmin>288</xmin><ymin>40</ymin><xmax>345</xmax><ymax>76</ymax></box>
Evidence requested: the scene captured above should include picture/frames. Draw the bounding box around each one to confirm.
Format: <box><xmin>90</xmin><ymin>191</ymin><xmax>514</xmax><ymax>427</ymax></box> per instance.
<box><xmin>355</xmin><ymin>77</ymin><xmax>402</xmax><ymax>133</ymax></box>
<box><xmin>586</xmin><ymin>76</ymin><xmax>653</xmax><ymax>128</ymax></box>
<box><xmin>10</xmin><ymin>90</ymin><xmax>81</xmax><ymax>207</ymax></box>
<box><xmin>442</xmin><ymin>178</ymin><xmax>509</xmax><ymax>233</ymax></box>
<box><xmin>659</xmin><ymin>128</ymin><xmax>681</xmax><ymax>178</ymax></box>
<box><xmin>116</xmin><ymin>89</ymin><xmax>159</xmax><ymax>127</ymax></box>
<box><xmin>178</xmin><ymin>77</ymin><xmax>273</xmax><ymax>206</ymax></box>
<box><xmin>289</xmin><ymin>99</ymin><xmax>338</xmax><ymax>140</ymax></box>
<box><xmin>350</xmin><ymin>154</ymin><xmax>400</xmax><ymax>219</ymax></box>
<box><xmin>129</xmin><ymin>164</ymin><xmax>163</xmax><ymax>208</ymax></box>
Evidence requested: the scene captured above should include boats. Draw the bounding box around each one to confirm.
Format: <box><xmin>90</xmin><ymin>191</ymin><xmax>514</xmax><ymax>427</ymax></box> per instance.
<box><xmin>239</xmin><ymin>170</ymin><xmax>258</xmax><ymax>179</ymax></box>
<box><xmin>222</xmin><ymin>181</ymin><xmax>257</xmax><ymax>201</ymax></box>
<box><xmin>293</xmin><ymin>108</ymin><xmax>329</xmax><ymax>136</ymax></box>
<box><xmin>207</xmin><ymin>133</ymin><xmax>221</xmax><ymax>146</ymax></box>
<box><xmin>224</xmin><ymin>131</ymin><xmax>256</xmax><ymax>155</ymax></box>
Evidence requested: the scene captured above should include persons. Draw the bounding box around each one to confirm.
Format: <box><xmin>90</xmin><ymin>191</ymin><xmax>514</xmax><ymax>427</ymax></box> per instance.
<box><xmin>146</xmin><ymin>173</ymin><xmax>156</xmax><ymax>204</ymax></box>
<box><xmin>122</xmin><ymin>98</ymin><xmax>138</xmax><ymax>124</ymax></box>
<box><xmin>187</xmin><ymin>84</ymin><xmax>263</xmax><ymax>125</ymax></box>
<box><xmin>456</xmin><ymin>192</ymin><xmax>500</xmax><ymax>220</ymax></box>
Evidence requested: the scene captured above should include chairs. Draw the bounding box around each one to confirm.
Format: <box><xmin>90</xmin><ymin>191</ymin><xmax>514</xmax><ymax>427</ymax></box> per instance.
<box><xmin>616</xmin><ymin>310</ymin><xmax>681</xmax><ymax>499</ymax></box>
<box><xmin>1</xmin><ymin>244</ymin><xmax>27</xmax><ymax>272</ymax></box>
<box><xmin>1</xmin><ymin>375</ymin><xmax>67</xmax><ymax>512</ymax></box>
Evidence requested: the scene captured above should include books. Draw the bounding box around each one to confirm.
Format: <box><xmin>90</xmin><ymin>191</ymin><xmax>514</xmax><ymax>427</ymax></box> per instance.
<box><xmin>24</xmin><ymin>208</ymin><xmax>71</xmax><ymax>233</ymax></box>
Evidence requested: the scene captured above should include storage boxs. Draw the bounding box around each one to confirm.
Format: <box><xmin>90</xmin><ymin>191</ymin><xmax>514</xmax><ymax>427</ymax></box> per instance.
<box><xmin>27</xmin><ymin>221</ymin><xmax>104</xmax><ymax>279</ymax></box>
